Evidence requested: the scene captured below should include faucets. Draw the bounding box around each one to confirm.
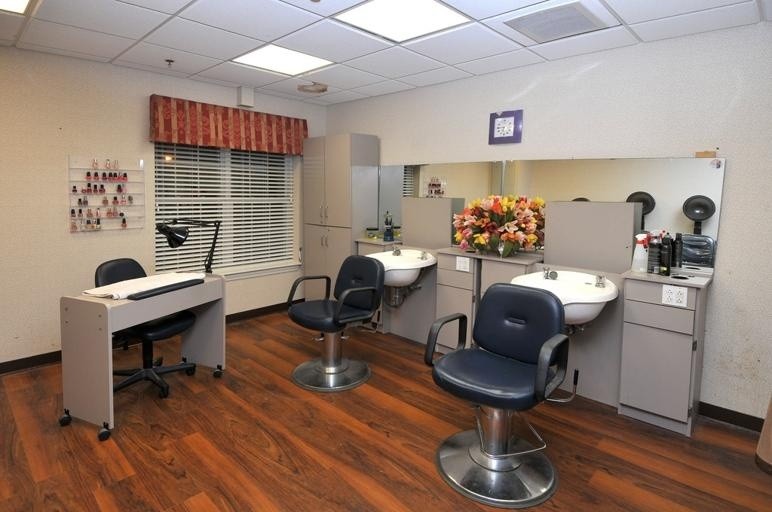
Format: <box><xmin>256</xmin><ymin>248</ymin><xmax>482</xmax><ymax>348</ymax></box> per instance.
<box><xmin>595</xmin><ymin>273</ymin><xmax>606</xmax><ymax>286</ymax></box>
<box><xmin>543</xmin><ymin>266</ymin><xmax>551</xmax><ymax>279</ymax></box>
<box><xmin>392</xmin><ymin>244</ymin><xmax>402</xmax><ymax>256</ymax></box>
<box><xmin>421</xmin><ymin>250</ymin><xmax>427</xmax><ymax>258</ymax></box>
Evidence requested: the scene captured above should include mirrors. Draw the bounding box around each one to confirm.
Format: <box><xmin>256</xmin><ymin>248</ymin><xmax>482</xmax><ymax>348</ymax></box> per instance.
<box><xmin>390</xmin><ymin>157</ymin><xmax>723</xmax><ymax>265</ymax></box>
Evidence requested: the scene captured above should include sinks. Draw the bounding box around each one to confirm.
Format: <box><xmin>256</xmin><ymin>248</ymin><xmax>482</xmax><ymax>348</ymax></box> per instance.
<box><xmin>365</xmin><ymin>250</ymin><xmax>437</xmax><ymax>287</ymax></box>
<box><xmin>511</xmin><ymin>271</ymin><xmax>619</xmax><ymax>325</ymax></box>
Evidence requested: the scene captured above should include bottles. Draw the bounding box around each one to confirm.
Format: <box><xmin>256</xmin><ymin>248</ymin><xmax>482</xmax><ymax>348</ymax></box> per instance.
<box><xmin>648</xmin><ymin>236</ymin><xmax>683</xmax><ymax>276</ymax></box>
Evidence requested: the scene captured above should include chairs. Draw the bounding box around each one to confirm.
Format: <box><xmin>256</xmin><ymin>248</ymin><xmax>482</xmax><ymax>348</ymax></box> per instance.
<box><xmin>92</xmin><ymin>257</ymin><xmax>199</xmax><ymax>398</ymax></box>
<box><xmin>422</xmin><ymin>282</ymin><xmax>582</xmax><ymax>509</ymax></box>
<box><xmin>282</xmin><ymin>255</ymin><xmax>386</xmax><ymax>393</ymax></box>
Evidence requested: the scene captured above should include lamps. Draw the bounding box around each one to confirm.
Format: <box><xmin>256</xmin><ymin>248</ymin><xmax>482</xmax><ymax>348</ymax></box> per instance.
<box><xmin>154</xmin><ymin>216</ymin><xmax>223</xmax><ymax>274</ymax></box>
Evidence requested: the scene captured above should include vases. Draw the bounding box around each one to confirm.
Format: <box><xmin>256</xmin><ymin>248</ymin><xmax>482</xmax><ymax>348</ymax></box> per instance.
<box><xmin>491</xmin><ymin>238</ymin><xmax>517</xmax><ymax>258</ymax></box>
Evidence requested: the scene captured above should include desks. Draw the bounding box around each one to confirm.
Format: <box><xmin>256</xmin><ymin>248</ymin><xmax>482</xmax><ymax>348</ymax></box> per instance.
<box><xmin>57</xmin><ymin>271</ymin><xmax>227</xmax><ymax>440</ymax></box>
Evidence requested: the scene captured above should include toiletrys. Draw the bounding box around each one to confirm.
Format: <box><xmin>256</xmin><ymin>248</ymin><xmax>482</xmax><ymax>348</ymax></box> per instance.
<box><xmin>631</xmin><ymin>228</ymin><xmax>683</xmax><ymax>276</ymax></box>
<box><xmin>384</xmin><ymin>226</ymin><xmax>394</xmax><ymax>240</ymax></box>
<box><xmin>70</xmin><ymin>158</ymin><xmax>133</xmax><ymax>232</ymax></box>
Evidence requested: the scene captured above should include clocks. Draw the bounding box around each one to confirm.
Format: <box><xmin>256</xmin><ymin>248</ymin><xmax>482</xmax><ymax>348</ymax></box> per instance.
<box><xmin>488</xmin><ymin>109</ymin><xmax>523</xmax><ymax>144</ymax></box>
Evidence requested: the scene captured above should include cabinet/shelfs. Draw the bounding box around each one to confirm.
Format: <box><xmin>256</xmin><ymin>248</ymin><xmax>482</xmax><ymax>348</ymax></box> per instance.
<box><xmin>618</xmin><ymin>272</ymin><xmax>711</xmax><ymax>437</ymax></box>
<box><xmin>302</xmin><ymin>132</ymin><xmax>378</xmax><ymax>303</ymax></box>
<box><xmin>431</xmin><ymin>247</ymin><xmax>534</xmax><ymax>357</ymax></box>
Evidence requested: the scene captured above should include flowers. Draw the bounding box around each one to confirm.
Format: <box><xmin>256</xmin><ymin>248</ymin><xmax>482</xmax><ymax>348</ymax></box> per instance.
<box><xmin>449</xmin><ymin>191</ymin><xmax>548</xmax><ymax>254</ymax></box>
